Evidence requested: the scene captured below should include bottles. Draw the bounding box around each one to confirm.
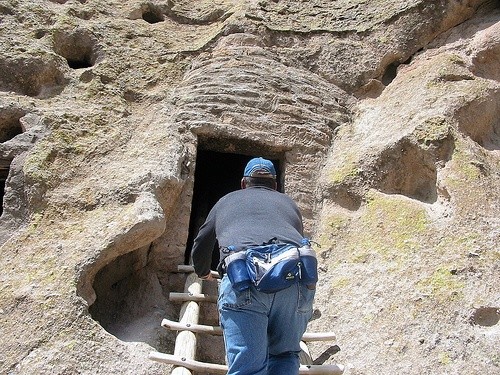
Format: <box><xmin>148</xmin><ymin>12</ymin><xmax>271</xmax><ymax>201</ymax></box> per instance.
<box><xmin>300</xmin><ymin>238</ymin><xmax>315</xmax><ymax>249</ymax></box>
<box><xmin>225</xmin><ymin>246</ymin><xmax>237</xmax><ymax>257</ymax></box>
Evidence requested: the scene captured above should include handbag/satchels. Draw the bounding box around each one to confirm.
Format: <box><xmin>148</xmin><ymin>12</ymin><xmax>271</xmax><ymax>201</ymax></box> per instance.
<box><xmin>224</xmin><ymin>236</ymin><xmax>319</xmax><ymax>296</ymax></box>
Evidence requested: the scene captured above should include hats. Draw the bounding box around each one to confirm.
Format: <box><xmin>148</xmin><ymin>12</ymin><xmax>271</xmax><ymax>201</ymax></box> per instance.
<box><xmin>244</xmin><ymin>157</ymin><xmax>276</xmax><ymax>179</ymax></box>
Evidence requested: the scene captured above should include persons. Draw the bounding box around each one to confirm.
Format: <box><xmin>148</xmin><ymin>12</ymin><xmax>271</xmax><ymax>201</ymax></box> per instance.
<box><xmin>192</xmin><ymin>157</ymin><xmax>318</xmax><ymax>375</ymax></box>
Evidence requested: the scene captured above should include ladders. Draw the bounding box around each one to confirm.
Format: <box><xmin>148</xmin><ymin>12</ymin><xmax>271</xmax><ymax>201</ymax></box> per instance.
<box><xmin>149</xmin><ymin>257</ymin><xmax>344</xmax><ymax>375</ymax></box>
